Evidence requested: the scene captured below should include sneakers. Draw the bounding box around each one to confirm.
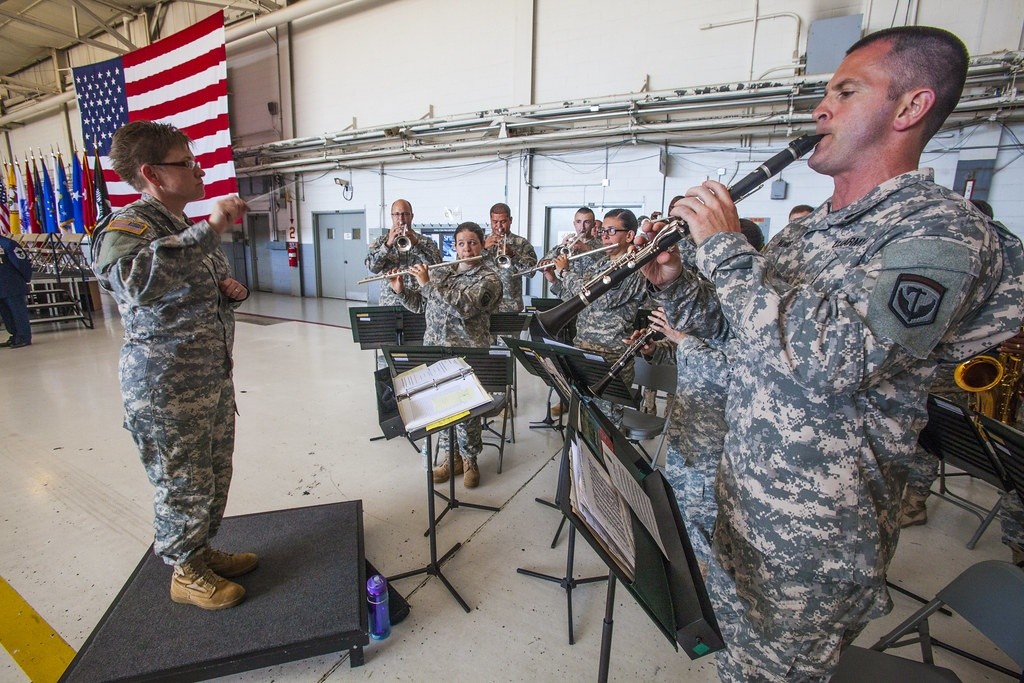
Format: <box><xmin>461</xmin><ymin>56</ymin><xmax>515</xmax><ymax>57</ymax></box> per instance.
<box><xmin>897</xmin><ymin>486</ymin><xmax>930</xmax><ymax>528</ymax></box>
<box><xmin>170</xmin><ymin>546</ymin><xmax>258</xmax><ymax>610</ymax></box>
<box><xmin>432</xmin><ymin>455</ymin><xmax>480</xmax><ymax>488</ymax></box>
<box><xmin>551</xmin><ymin>401</ymin><xmax>568</xmax><ymax>416</ymax></box>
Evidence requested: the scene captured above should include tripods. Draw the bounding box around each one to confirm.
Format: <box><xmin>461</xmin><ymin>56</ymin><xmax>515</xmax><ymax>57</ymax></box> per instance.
<box><xmin>350</xmin><ymin>298</ymin><xmax>653</xmax><ymax>646</ymax></box>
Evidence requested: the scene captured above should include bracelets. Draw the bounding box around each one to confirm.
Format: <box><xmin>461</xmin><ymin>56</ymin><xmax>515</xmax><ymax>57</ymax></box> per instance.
<box><xmin>558</xmin><ymin>268</ymin><xmax>570</xmax><ymax>278</ymax></box>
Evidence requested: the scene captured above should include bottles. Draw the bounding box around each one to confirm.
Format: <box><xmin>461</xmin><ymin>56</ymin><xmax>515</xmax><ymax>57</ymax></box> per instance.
<box><xmin>366</xmin><ymin>574</ymin><xmax>391</xmax><ymax>640</ymax></box>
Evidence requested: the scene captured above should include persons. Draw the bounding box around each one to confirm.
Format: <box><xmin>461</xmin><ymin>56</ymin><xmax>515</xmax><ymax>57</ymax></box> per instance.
<box><xmin>621</xmin><ymin>24</ymin><xmax>1001</xmax><ymax>683</ymax></box>
<box><xmin>0</xmin><ymin>236</ymin><xmax>33</xmax><ymax>348</ymax></box>
<box><xmin>480</xmin><ymin>203</ymin><xmax>538</xmax><ymax>419</ymax></box>
<box><xmin>364</xmin><ymin>198</ymin><xmax>443</xmax><ymax>370</ymax></box>
<box><xmin>899</xmin><ymin>197</ymin><xmax>1024</xmax><ymax>569</ymax></box>
<box><xmin>88</xmin><ymin>119</ymin><xmax>259</xmax><ymax>611</ymax></box>
<box><xmin>537</xmin><ymin>195</ymin><xmax>815</xmax><ymax>442</ymax></box>
<box><xmin>387</xmin><ymin>221</ymin><xmax>504</xmax><ymax>489</ymax></box>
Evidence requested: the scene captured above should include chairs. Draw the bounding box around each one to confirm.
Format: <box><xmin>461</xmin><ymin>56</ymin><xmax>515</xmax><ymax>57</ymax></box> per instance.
<box><xmin>480</xmin><ymin>347</ymin><xmax>1024</xmax><ymax>683</ymax></box>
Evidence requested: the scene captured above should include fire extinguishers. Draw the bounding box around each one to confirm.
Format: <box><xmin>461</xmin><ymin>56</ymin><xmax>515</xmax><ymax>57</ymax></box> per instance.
<box><xmin>287</xmin><ymin>243</ymin><xmax>297</xmax><ymax>267</ymax></box>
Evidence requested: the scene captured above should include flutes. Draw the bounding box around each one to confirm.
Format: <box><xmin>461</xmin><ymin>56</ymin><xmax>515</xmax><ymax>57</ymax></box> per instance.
<box><xmin>511</xmin><ymin>242</ymin><xmax>621</xmax><ymax>279</ymax></box>
<box><xmin>532</xmin><ymin>132</ymin><xmax>827</xmax><ymax>341</ymax></box>
<box><xmin>585</xmin><ymin>319</ymin><xmax>659</xmax><ymax>399</ymax></box>
<box><xmin>357</xmin><ymin>254</ymin><xmax>486</xmax><ymax>286</ymax></box>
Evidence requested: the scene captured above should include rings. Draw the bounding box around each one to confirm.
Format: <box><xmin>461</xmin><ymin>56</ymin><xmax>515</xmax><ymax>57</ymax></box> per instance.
<box><xmin>235</xmin><ymin>288</ymin><xmax>240</xmax><ymax>293</ymax></box>
<box><xmin>416</xmin><ymin>270</ymin><xmax>420</xmax><ymax>274</ymax></box>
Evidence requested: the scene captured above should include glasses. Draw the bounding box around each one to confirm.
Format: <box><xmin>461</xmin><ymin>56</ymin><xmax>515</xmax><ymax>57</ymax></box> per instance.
<box><xmin>139</xmin><ymin>161</ymin><xmax>202</xmax><ymax>171</ymax></box>
<box><xmin>597</xmin><ymin>228</ymin><xmax>629</xmax><ymax>237</ymax></box>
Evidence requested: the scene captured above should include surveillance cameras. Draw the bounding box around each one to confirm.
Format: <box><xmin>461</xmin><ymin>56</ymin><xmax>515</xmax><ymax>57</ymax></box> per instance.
<box><xmin>334</xmin><ymin>177</ymin><xmax>349</xmax><ymax>186</ymax></box>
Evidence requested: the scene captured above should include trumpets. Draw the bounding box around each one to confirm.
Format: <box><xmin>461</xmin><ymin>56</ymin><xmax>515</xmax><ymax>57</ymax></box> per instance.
<box><xmin>495</xmin><ymin>227</ymin><xmax>513</xmax><ymax>271</ymax></box>
<box><xmin>635</xmin><ymin>213</ymin><xmax>674</xmax><ymax>234</ymax></box>
<box><xmin>394</xmin><ymin>223</ymin><xmax>413</xmax><ymax>255</ymax></box>
<box><xmin>559</xmin><ymin>230</ymin><xmax>589</xmax><ymax>257</ymax></box>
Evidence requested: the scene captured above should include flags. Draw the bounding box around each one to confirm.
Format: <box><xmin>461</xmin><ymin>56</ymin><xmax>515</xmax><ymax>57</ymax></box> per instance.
<box><xmin>69</xmin><ymin>9</ymin><xmax>244</xmax><ymax>226</ymax></box>
<box><xmin>0</xmin><ymin>150</ymin><xmax>112</xmax><ymax>235</ymax></box>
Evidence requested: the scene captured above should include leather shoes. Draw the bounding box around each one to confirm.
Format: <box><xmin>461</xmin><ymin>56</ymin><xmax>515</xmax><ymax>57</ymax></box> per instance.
<box><xmin>0</xmin><ymin>338</ymin><xmax>31</xmax><ymax>348</ymax></box>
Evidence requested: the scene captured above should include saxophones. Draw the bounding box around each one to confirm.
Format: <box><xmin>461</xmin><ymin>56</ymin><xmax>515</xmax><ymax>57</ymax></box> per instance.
<box><xmin>953</xmin><ymin>322</ymin><xmax>1024</xmax><ymax>436</ymax></box>
